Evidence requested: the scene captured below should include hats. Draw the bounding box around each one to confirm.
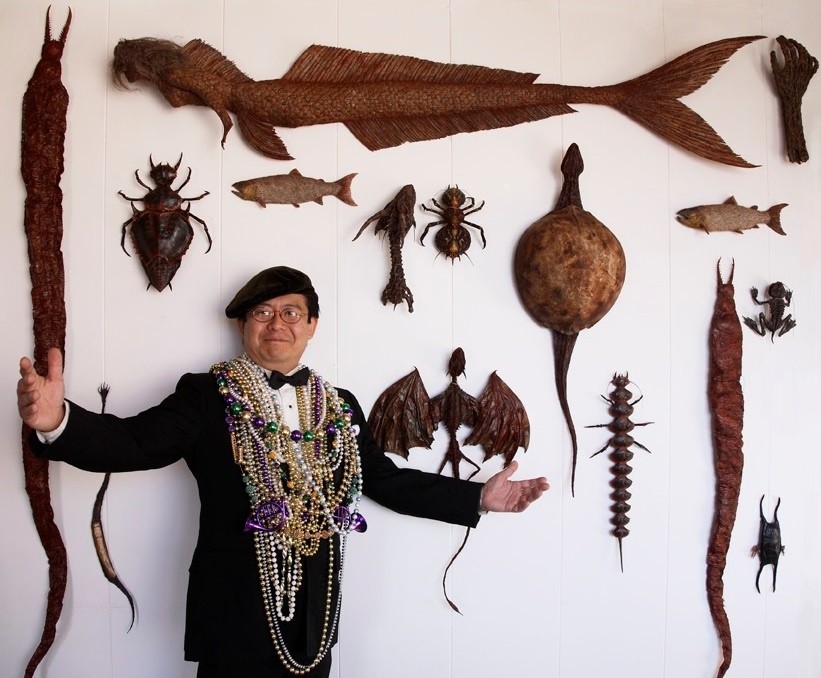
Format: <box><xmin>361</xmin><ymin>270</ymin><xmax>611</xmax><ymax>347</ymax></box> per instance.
<box><xmin>225</xmin><ymin>266</ymin><xmax>315</xmax><ymax>318</ymax></box>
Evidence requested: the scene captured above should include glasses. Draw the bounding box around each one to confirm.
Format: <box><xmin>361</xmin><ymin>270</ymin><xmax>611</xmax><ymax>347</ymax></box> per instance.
<box><xmin>248</xmin><ymin>307</ymin><xmax>309</xmax><ymax>324</ymax></box>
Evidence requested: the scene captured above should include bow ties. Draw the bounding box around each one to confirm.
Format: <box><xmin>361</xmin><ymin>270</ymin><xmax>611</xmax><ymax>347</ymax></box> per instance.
<box><xmin>264</xmin><ymin>366</ymin><xmax>310</xmax><ymax>390</ymax></box>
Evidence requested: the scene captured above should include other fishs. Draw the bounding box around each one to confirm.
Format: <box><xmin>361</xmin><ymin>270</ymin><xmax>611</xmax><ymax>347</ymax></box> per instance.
<box><xmin>110</xmin><ymin>33</ymin><xmax>770</xmax><ymax>211</ymax></box>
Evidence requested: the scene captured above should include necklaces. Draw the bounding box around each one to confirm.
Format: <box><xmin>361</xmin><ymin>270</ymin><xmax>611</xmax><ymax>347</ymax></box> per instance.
<box><xmin>210</xmin><ymin>352</ymin><xmax>363</xmax><ymax>672</ymax></box>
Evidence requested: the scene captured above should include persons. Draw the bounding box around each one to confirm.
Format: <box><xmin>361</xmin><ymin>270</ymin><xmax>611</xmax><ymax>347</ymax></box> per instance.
<box><xmin>15</xmin><ymin>265</ymin><xmax>551</xmax><ymax>678</ymax></box>
